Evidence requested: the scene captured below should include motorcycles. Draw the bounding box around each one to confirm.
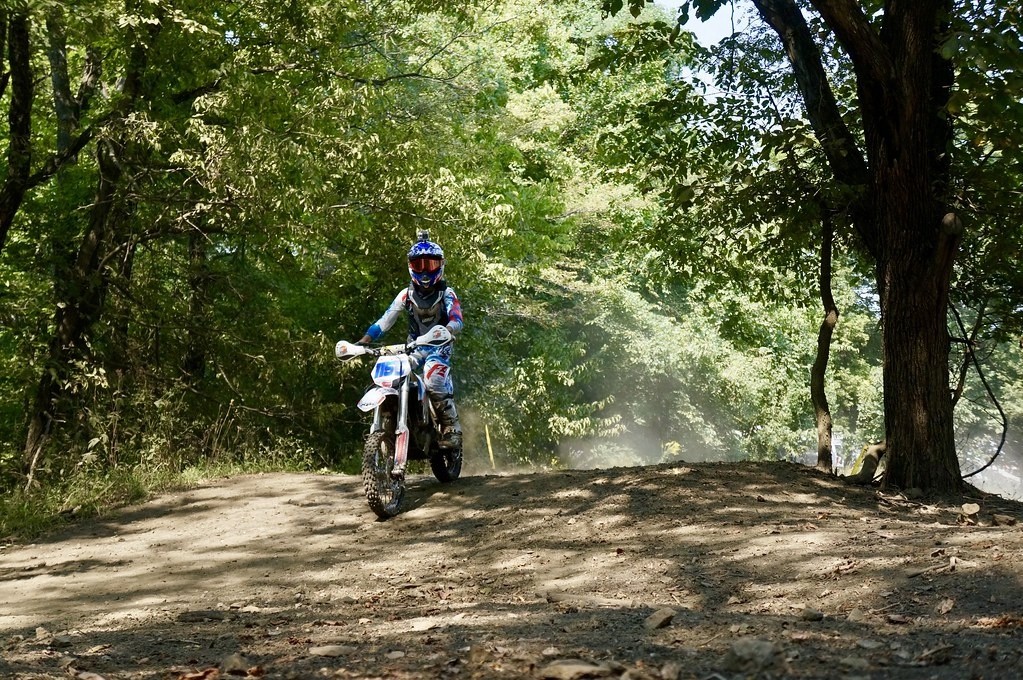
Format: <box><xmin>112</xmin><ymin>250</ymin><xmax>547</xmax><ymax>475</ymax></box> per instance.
<box><xmin>332</xmin><ymin>325</ymin><xmax>463</xmax><ymax>521</ymax></box>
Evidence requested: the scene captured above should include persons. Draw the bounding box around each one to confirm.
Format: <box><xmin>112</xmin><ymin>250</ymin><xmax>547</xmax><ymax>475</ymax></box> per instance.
<box><xmin>353</xmin><ymin>242</ymin><xmax>465</xmax><ymax>449</ymax></box>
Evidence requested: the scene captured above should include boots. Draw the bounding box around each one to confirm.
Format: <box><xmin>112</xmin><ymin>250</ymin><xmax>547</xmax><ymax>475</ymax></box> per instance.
<box><xmin>430</xmin><ymin>394</ymin><xmax>463</xmax><ymax>449</ymax></box>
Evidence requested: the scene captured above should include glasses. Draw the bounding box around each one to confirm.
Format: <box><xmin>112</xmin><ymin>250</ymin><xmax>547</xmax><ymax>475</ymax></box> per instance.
<box><xmin>409</xmin><ymin>259</ymin><xmax>442</xmax><ymax>273</ymax></box>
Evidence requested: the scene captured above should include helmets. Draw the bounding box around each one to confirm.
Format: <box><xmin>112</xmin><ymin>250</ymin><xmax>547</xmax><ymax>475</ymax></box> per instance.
<box><xmin>408</xmin><ymin>241</ymin><xmax>445</xmax><ymax>287</ymax></box>
